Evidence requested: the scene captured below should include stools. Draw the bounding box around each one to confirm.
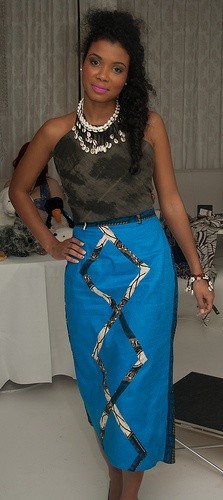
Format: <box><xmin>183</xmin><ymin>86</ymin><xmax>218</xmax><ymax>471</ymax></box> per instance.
<box><xmin>173</xmin><ymin>372</ymin><xmax>223</xmax><ymax>473</ymax></box>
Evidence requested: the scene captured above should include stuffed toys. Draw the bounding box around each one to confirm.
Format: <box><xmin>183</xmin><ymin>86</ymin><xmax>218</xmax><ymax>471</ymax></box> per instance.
<box><xmin>0</xmin><ymin>186</ymin><xmax>73</xmax><ymax>257</ymax></box>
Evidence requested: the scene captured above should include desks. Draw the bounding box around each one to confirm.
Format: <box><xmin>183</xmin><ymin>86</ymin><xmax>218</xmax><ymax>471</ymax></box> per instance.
<box><xmin>0</xmin><ymin>253</ymin><xmax>223</xmax><ymax>393</ymax></box>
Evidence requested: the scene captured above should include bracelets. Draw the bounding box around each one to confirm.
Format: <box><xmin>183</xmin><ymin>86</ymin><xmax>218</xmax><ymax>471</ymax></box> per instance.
<box><xmin>185</xmin><ymin>274</ymin><xmax>214</xmax><ymax>294</ymax></box>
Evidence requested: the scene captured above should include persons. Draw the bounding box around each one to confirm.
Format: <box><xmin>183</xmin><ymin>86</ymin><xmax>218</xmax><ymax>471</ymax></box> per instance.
<box><xmin>11</xmin><ymin>142</ymin><xmax>72</xmax><ymax>230</ymax></box>
<box><xmin>8</xmin><ymin>9</ymin><xmax>216</xmax><ymax>500</ymax></box>
